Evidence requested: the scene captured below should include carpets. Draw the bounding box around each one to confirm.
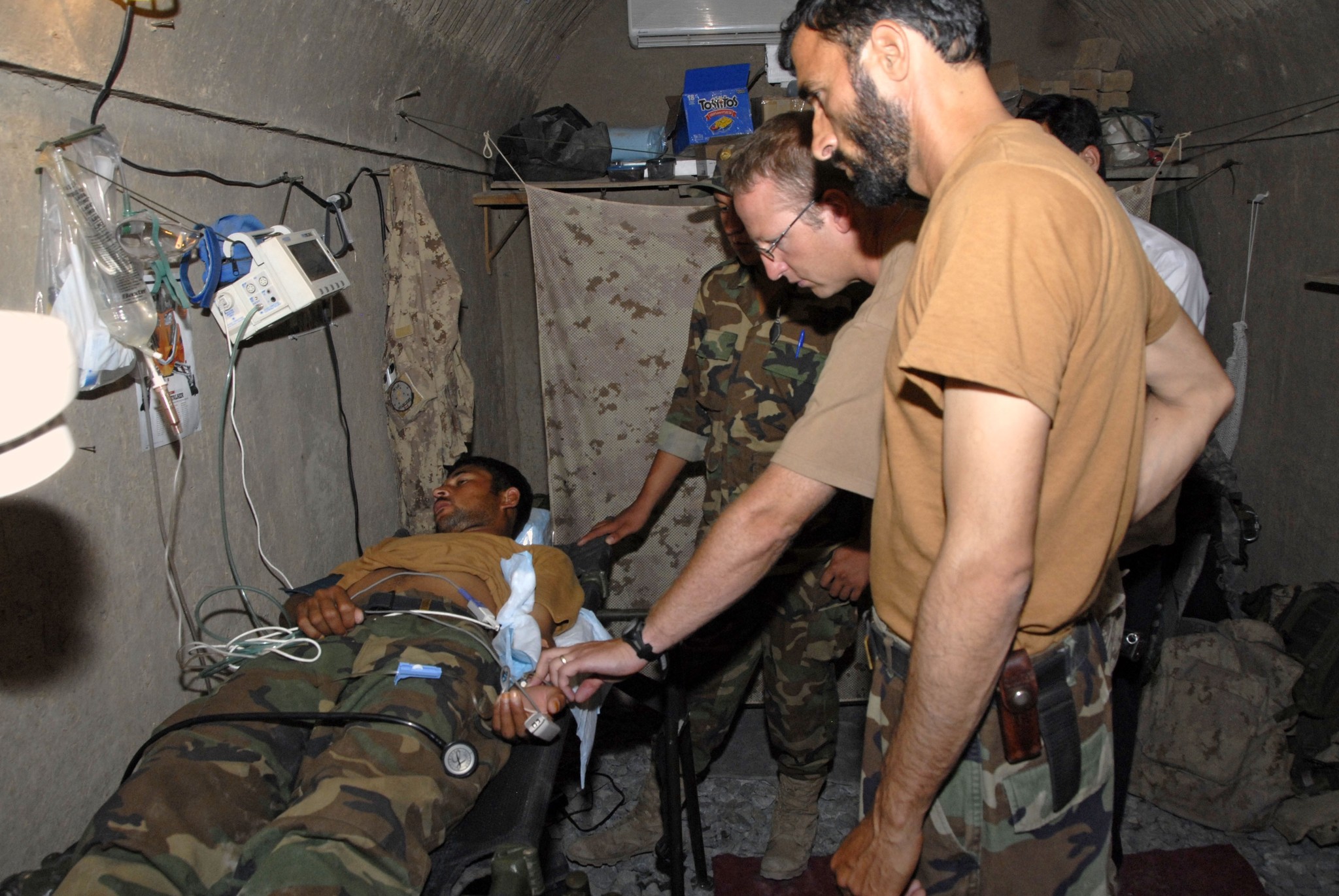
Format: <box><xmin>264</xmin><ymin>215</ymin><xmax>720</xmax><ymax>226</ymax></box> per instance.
<box><xmin>710</xmin><ymin>843</ymin><xmax>1270</xmax><ymax>896</ymax></box>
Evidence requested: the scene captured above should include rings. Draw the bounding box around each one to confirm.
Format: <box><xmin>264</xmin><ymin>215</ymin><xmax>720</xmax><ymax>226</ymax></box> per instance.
<box><xmin>559</xmin><ymin>655</ymin><xmax>568</xmax><ymax>666</ymax></box>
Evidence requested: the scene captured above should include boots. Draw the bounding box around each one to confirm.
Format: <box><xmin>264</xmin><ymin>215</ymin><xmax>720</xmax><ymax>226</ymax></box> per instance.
<box><xmin>564</xmin><ymin>770</ymin><xmax>686</xmax><ymax>868</ymax></box>
<box><xmin>758</xmin><ymin>774</ymin><xmax>825</xmax><ymax>880</ymax></box>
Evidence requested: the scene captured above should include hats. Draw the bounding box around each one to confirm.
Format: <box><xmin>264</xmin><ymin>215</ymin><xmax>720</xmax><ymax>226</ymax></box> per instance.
<box><xmin>689</xmin><ymin>134</ymin><xmax>756</xmax><ymax>197</ymax></box>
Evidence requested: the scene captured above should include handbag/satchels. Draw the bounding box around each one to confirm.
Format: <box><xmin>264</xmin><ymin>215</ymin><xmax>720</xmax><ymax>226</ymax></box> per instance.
<box><xmin>29</xmin><ymin>156</ymin><xmax>139</xmax><ymax>394</ymax></box>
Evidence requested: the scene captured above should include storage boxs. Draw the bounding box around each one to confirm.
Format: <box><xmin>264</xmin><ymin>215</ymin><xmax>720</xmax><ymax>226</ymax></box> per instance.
<box><xmin>663</xmin><ymin>61</ymin><xmax>752</xmax><ymax>156</ymax></box>
<box><xmin>750</xmin><ymin>96</ymin><xmax>813</xmax><ymax>130</ymax></box>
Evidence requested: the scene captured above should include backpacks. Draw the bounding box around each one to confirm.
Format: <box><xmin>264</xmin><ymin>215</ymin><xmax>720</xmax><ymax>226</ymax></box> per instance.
<box><xmin>1099</xmin><ymin>438</ymin><xmax>1338</xmax><ymax>896</ymax></box>
<box><xmin>495</xmin><ymin>102</ymin><xmax>613</xmax><ymax>183</ymax></box>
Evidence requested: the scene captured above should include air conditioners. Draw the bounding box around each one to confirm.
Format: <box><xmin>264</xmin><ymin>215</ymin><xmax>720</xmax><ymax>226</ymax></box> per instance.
<box><xmin>626</xmin><ymin>0</ymin><xmax>798</xmax><ymax>50</ymax></box>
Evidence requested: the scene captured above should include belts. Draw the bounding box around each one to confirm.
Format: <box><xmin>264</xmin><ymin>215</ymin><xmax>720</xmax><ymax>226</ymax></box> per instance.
<box><xmin>865</xmin><ymin>625</ymin><xmax>1092</xmax><ymax>703</ymax></box>
<box><xmin>362</xmin><ymin>590</ymin><xmax>475</xmax><ymax>619</ymax></box>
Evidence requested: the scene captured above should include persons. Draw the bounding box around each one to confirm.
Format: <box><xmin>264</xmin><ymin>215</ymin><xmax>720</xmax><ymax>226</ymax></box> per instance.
<box><xmin>532</xmin><ymin>0</ymin><xmax>1235</xmax><ymax>896</ymax></box>
<box><xmin>0</xmin><ymin>455</ymin><xmax>584</xmax><ymax>896</ymax></box>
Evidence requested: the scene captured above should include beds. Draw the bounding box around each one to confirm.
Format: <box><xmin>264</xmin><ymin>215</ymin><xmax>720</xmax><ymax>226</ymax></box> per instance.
<box><xmin>0</xmin><ymin>516</ymin><xmax>710</xmax><ymax>896</ymax></box>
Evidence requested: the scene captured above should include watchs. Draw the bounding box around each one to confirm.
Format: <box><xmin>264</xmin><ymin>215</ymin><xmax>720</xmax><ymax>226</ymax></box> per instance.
<box><xmin>622</xmin><ymin>617</ymin><xmax>662</xmax><ymax>661</ymax></box>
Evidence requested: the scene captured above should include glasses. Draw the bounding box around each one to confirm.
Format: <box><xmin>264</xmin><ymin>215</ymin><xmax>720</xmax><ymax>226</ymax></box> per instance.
<box><xmin>750</xmin><ymin>201</ymin><xmax>814</xmax><ymax>262</ymax></box>
